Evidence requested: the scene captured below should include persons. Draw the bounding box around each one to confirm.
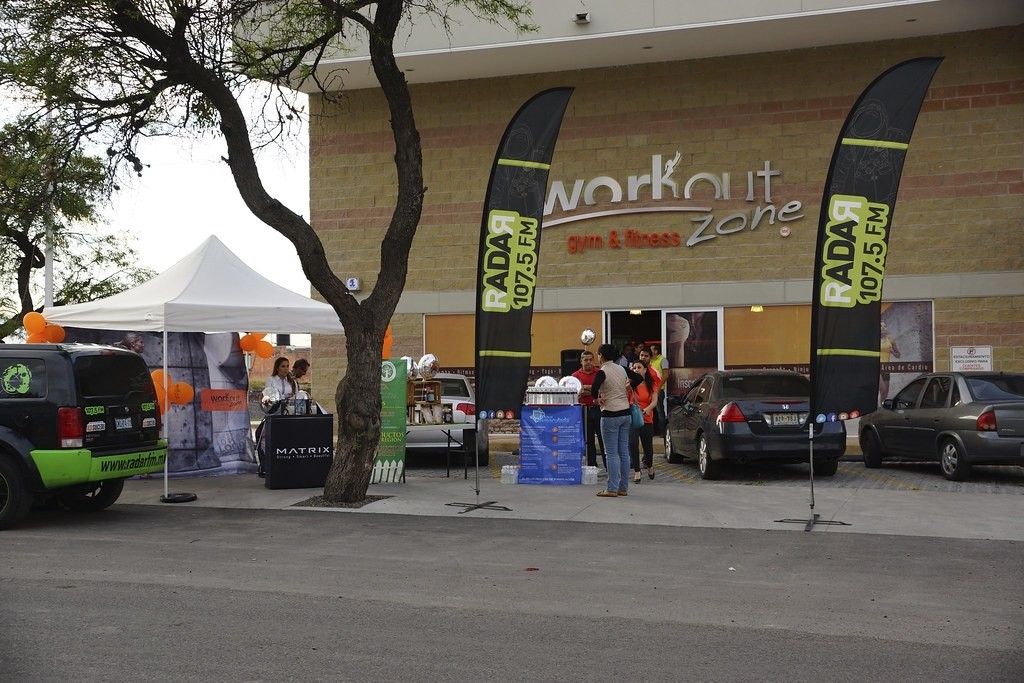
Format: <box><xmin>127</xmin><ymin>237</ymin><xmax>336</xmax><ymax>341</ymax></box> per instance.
<box><xmin>265</xmin><ymin>357</ymin><xmax>297</xmax><ymax>397</ymax></box>
<box><xmin>290</xmin><ymin>358</ymin><xmax>309</xmax><ymax>390</ymax></box>
<box><xmin>614</xmin><ymin>340</ymin><xmax>669</xmax><ymax>484</ymax></box>
<box><xmin>112</xmin><ymin>332</ymin><xmax>144</xmax><ymax>353</ymax></box>
<box><xmin>573</xmin><ymin>351</ymin><xmax>606</xmax><ymax>469</ymax></box>
<box><xmin>592</xmin><ymin>344</ymin><xmax>643</xmax><ymax>497</ymax></box>
<box><xmin>878</xmin><ymin>320</ymin><xmax>900</xmax><ymax>403</ymax></box>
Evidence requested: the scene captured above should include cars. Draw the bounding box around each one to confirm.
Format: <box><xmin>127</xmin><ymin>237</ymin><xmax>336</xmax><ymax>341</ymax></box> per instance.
<box><xmin>665</xmin><ymin>371</ymin><xmax>847</xmax><ymax>479</ymax></box>
<box><xmin>407</xmin><ymin>374</ymin><xmax>490</xmax><ymax>466</ymax></box>
<box><xmin>857</xmin><ymin>371</ymin><xmax>1024</xmax><ymax>480</ymax></box>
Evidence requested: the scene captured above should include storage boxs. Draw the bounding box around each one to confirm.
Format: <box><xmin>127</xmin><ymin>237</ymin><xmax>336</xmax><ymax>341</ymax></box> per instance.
<box><xmin>407</xmin><ymin>380</ymin><xmax>454</xmax><ymax>422</ymax></box>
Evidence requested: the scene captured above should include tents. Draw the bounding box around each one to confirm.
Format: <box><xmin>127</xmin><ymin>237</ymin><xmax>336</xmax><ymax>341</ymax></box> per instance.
<box><xmin>42</xmin><ymin>235</ymin><xmax>343</xmax><ymax>497</ymax></box>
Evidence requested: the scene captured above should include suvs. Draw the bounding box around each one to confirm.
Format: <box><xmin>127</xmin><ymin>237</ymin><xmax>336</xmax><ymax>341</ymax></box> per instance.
<box><xmin>0</xmin><ymin>344</ymin><xmax>169</xmax><ymax>532</ymax></box>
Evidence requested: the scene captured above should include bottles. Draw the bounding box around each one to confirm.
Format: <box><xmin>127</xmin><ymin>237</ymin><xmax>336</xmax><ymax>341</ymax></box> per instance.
<box><xmin>501</xmin><ymin>466</ymin><xmax>517</xmax><ymax>485</ymax></box>
<box><xmin>582</xmin><ymin>466</ymin><xmax>598</xmax><ymax>485</ymax></box>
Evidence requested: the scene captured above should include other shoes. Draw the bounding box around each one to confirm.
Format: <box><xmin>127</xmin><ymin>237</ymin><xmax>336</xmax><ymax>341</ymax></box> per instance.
<box><xmin>617</xmin><ymin>490</ymin><xmax>627</xmax><ymax>495</ymax></box>
<box><xmin>642</xmin><ymin>458</ymin><xmax>648</xmax><ymax>469</ymax></box>
<box><xmin>597</xmin><ymin>489</ymin><xmax>618</xmax><ymax>497</ymax></box>
<box><xmin>648</xmin><ymin>467</ymin><xmax>655</xmax><ymax>480</ymax></box>
<box><xmin>634</xmin><ymin>471</ymin><xmax>643</xmax><ymax>483</ymax></box>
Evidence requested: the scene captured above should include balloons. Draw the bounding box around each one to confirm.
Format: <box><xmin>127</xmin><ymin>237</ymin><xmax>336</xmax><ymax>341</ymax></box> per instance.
<box><xmin>170</xmin><ymin>382</ymin><xmax>194</xmax><ymax>405</ymax></box>
<box><xmin>240</xmin><ymin>335</ymin><xmax>257</xmax><ymax>351</ymax></box>
<box><xmin>383</xmin><ymin>324</ymin><xmax>392</xmax><ymax>359</ymax></box>
<box><xmin>256</xmin><ymin>341</ymin><xmax>274</xmax><ymax>358</ymax></box>
<box><xmin>251</xmin><ymin>333</ymin><xmax>267</xmax><ymax>340</ymax></box>
<box><xmin>151</xmin><ymin>370</ymin><xmax>171</xmax><ymax>414</ymax></box>
<box><xmin>401</xmin><ymin>356</ymin><xmax>419</xmax><ymax>380</ymax></box>
<box><xmin>23</xmin><ymin>312</ymin><xmax>65</xmax><ymax>343</ymax></box>
<box><xmin>419</xmin><ymin>353</ymin><xmax>439</xmax><ymax>382</ymax></box>
<box><xmin>260</xmin><ymin>387</ymin><xmax>282</xmax><ymax>415</ymax></box>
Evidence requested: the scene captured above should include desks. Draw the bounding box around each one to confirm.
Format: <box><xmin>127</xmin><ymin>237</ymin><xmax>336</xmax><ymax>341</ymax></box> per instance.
<box><xmin>263</xmin><ymin>413</ymin><xmax>334</xmax><ymax>490</ymax></box>
<box><xmin>518</xmin><ymin>404</ymin><xmax>588</xmax><ymax>484</ymax></box>
<box><xmin>404</xmin><ymin>423</ymin><xmax>480</xmax><ymax>495</ymax></box>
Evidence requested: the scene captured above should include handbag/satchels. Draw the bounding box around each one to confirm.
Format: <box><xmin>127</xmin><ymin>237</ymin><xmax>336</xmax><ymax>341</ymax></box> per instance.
<box><xmin>629</xmin><ymin>404</ymin><xmax>645</xmax><ymax>428</ymax></box>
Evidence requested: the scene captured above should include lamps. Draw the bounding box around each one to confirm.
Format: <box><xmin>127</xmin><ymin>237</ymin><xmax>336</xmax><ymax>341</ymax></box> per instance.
<box><xmin>629</xmin><ymin>310</ymin><xmax>642</xmax><ymax>314</ymax></box>
<box><xmin>750</xmin><ymin>305</ymin><xmax>764</xmax><ymax>312</ymax></box>
